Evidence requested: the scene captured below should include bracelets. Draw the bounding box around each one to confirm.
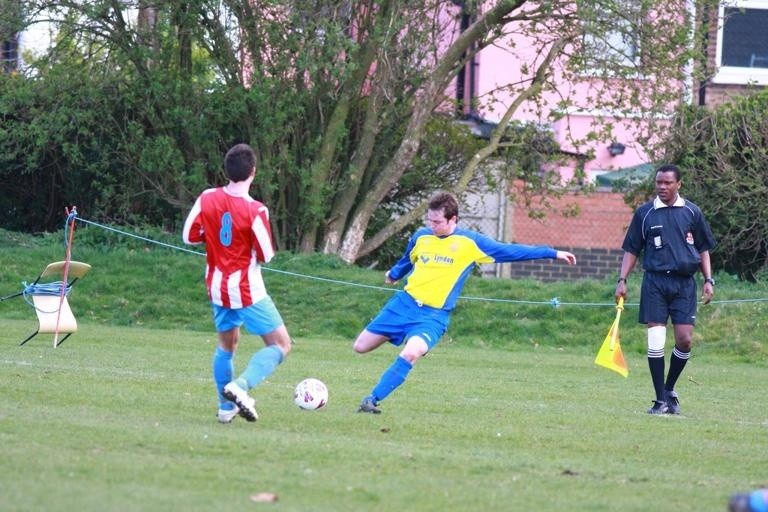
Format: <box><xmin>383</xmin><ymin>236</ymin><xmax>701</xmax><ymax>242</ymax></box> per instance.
<box><xmin>617</xmin><ymin>277</ymin><xmax>627</xmax><ymax>283</ymax></box>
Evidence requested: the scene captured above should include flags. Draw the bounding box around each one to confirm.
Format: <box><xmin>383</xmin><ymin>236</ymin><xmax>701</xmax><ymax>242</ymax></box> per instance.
<box><xmin>595</xmin><ymin>314</ymin><xmax>629</xmax><ymax>378</ymax></box>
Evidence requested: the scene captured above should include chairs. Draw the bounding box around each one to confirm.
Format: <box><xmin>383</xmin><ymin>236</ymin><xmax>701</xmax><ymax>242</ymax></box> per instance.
<box><xmin>0</xmin><ymin>261</ymin><xmax>93</xmax><ymax>347</ymax></box>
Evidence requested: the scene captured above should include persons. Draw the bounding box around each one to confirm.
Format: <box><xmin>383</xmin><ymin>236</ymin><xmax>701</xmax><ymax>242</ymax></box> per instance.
<box><xmin>183</xmin><ymin>143</ymin><xmax>291</xmax><ymax>423</ymax></box>
<box><xmin>615</xmin><ymin>166</ymin><xmax>716</xmax><ymax>415</ymax></box>
<box><xmin>354</xmin><ymin>190</ymin><xmax>577</xmax><ymax>412</ymax></box>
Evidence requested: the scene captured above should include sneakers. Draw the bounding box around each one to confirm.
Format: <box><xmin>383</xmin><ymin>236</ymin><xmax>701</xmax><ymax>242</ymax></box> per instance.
<box><xmin>664</xmin><ymin>386</ymin><xmax>681</xmax><ymax>415</ymax></box>
<box><xmin>647</xmin><ymin>398</ymin><xmax>668</xmax><ymax>415</ymax></box>
<box><xmin>358</xmin><ymin>400</ymin><xmax>380</xmax><ymax>414</ymax></box>
<box><xmin>217</xmin><ymin>382</ymin><xmax>258</xmax><ymax>424</ymax></box>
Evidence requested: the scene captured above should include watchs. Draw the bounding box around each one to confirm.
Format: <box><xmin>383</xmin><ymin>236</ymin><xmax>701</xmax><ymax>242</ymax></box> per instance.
<box><xmin>704</xmin><ymin>277</ymin><xmax>715</xmax><ymax>286</ymax></box>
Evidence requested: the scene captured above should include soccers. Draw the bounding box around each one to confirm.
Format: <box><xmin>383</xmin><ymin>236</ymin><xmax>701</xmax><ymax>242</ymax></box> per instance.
<box><xmin>294</xmin><ymin>378</ymin><xmax>328</xmax><ymax>411</ymax></box>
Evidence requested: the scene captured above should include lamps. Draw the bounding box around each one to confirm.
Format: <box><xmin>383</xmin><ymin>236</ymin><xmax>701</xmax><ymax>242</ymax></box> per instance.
<box><xmin>609</xmin><ymin>142</ymin><xmax>626</xmax><ymax>157</ymax></box>
